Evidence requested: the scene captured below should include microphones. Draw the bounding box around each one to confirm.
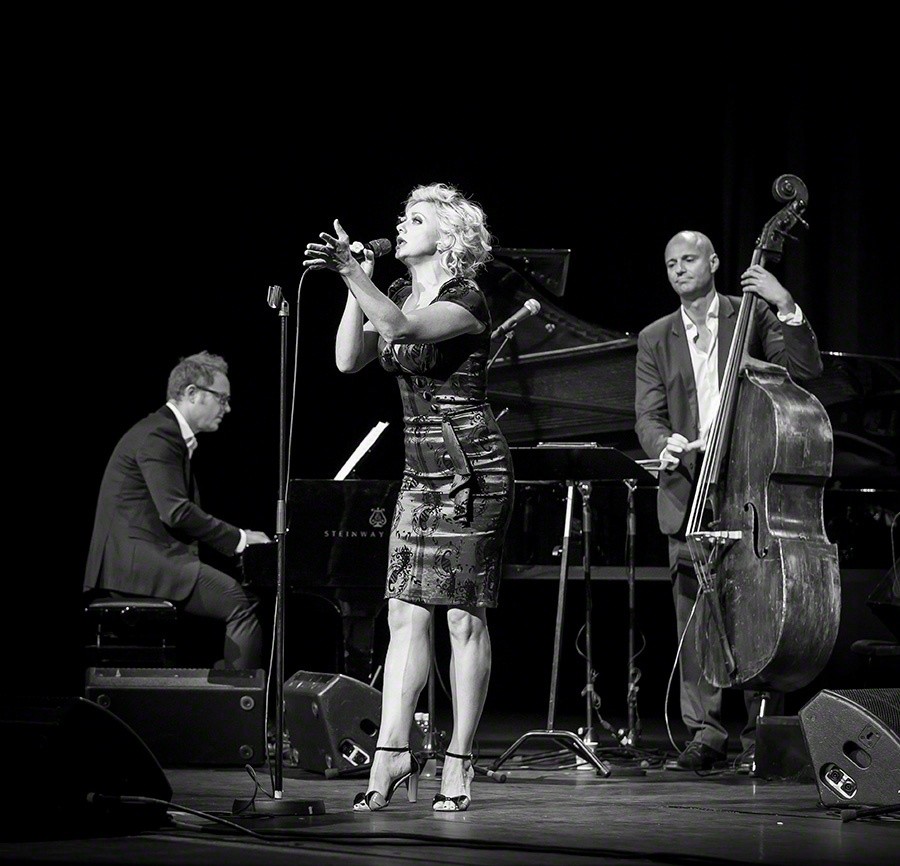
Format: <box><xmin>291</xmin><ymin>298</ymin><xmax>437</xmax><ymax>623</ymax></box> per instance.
<box><xmin>490</xmin><ymin>299</ymin><xmax>540</xmax><ymax>340</ymax></box>
<box><xmin>350</xmin><ymin>238</ymin><xmax>392</xmax><ymax>264</ymax></box>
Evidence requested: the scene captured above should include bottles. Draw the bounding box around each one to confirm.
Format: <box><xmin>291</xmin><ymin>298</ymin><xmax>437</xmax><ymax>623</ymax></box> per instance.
<box><xmin>415</xmin><ymin>713</ymin><xmax>424</xmax><ymax>726</ymax></box>
<box><xmin>420</xmin><ymin>713</ymin><xmax>435</xmax><ymax>775</ymax></box>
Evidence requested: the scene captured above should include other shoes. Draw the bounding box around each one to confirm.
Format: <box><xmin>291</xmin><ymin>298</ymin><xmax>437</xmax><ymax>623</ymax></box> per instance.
<box><xmin>738</xmin><ymin>752</ymin><xmax>755</xmax><ymax>773</ymax></box>
<box><xmin>677</xmin><ymin>742</ymin><xmax>717</xmax><ymax>771</ymax></box>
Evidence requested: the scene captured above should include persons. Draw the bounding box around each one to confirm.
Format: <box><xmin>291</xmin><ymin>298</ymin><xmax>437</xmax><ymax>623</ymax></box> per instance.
<box><xmin>82</xmin><ymin>350</ymin><xmax>273</xmax><ymax>674</ymax></box>
<box><xmin>302</xmin><ymin>183</ymin><xmax>514</xmax><ymax>813</ymax></box>
<box><xmin>634</xmin><ymin>230</ymin><xmax>823</xmax><ymax>774</ymax></box>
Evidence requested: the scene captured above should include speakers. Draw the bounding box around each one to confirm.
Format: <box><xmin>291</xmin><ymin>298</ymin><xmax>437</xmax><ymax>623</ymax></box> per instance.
<box><xmin>61</xmin><ymin>696</ymin><xmax>173</xmax><ymax>818</ymax></box>
<box><xmin>798</xmin><ymin>688</ymin><xmax>900</xmax><ymax>807</ymax></box>
<box><xmin>282</xmin><ymin>670</ymin><xmax>427</xmax><ymax>778</ymax></box>
<box><xmin>86</xmin><ymin>667</ymin><xmax>267</xmax><ymax>767</ymax></box>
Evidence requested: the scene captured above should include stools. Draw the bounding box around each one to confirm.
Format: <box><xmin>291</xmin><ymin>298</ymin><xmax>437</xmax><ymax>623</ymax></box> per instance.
<box><xmin>80</xmin><ymin>599</ymin><xmax>183</xmax><ymax>663</ymax></box>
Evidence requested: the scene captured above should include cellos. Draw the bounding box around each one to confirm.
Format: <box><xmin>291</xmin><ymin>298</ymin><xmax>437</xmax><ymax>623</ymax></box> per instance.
<box><xmin>684</xmin><ymin>174</ymin><xmax>843</xmax><ymax>772</ymax></box>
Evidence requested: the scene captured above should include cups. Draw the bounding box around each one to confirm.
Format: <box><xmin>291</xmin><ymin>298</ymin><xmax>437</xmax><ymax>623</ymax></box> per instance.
<box><xmin>574</xmin><ymin>742</ymin><xmax>599</xmax><ymax>770</ymax></box>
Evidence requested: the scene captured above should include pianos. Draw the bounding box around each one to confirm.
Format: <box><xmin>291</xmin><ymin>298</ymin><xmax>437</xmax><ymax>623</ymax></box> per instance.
<box><xmin>241</xmin><ymin>247</ymin><xmax>900</xmax><ymax>692</ymax></box>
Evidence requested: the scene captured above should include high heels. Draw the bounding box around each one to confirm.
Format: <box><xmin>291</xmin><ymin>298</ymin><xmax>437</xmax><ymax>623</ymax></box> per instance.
<box><xmin>432</xmin><ymin>751</ymin><xmax>475</xmax><ymax>811</ymax></box>
<box><xmin>353</xmin><ymin>746</ymin><xmax>423</xmax><ymax>812</ymax></box>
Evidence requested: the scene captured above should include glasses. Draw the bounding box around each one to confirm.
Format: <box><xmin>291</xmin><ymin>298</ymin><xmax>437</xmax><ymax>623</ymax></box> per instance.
<box><xmin>185</xmin><ymin>383</ymin><xmax>232</xmax><ymax>405</ymax></box>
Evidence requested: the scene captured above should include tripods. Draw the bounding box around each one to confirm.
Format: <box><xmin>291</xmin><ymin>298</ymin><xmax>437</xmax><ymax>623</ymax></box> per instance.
<box><xmin>325</xmin><ymin>448</ymin><xmax>663</xmax><ymax>783</ymax></box>
